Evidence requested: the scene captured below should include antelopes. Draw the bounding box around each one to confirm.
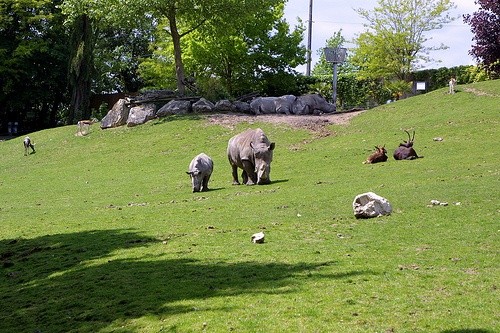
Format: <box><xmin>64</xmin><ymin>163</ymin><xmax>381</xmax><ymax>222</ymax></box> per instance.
<box><xmin>23</xmin><ymin>136</ymin><xmax>36</xmax><ymax>155</ymax></box>
<box><xmin>394</xmin><ymin>130</ymin><xmax>425</xmax><ymax>159</ymax></box>
<box><xmin>367</xmin><ymin>144</ymin><xmax>388</xmax><ymax>163</ymax></box>
